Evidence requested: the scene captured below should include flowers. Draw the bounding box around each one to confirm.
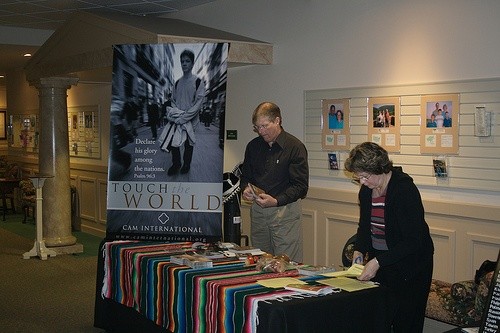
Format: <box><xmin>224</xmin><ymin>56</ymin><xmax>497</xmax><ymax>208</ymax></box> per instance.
<box><xmin>19</xmin><ymin>179</ymin><xmax>36</xmax><ymax>201</ymax></box>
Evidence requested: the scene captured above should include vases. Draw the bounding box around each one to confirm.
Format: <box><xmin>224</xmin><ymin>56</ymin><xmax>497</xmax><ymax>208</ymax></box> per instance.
<box><xmin>25</xmin><ymin>199</ymin><xmax>34</xmax><ymax>208</ymax></box>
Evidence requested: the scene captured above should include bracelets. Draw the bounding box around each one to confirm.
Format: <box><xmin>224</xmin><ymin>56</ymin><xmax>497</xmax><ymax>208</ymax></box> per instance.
<box><xmin>373</xmin><ymin>256</ymin><xmax>380</xmax><ymax>268</ymax></box>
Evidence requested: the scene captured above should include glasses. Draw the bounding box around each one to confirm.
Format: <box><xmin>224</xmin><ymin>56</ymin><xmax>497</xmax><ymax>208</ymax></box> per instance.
<box><xmin>353</xmin><ymin>172</ymin><xmax>371</xmax><ymax>181</ymax></box>
<box><xmin>253</xmin><ymin>120</ymin><xmax>273</xmax><ymax>129</ymax></box>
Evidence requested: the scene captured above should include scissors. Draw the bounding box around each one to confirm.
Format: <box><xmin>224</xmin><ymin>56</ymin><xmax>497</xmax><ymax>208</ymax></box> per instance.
<box><xmin>345</xmin><ymin>257</ymin><xmax>361</xmax><ymax>272</ymax></box>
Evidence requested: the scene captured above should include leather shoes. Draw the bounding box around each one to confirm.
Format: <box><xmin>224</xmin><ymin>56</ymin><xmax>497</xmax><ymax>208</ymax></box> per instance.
<box><xmin>165</xmin><ymin>162</ymin><xmax>193</xmax><ymax>175</ymax></box>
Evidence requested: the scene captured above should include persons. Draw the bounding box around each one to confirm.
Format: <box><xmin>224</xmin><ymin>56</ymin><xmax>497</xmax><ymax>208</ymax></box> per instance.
<box><xmin>158</xmin><ymin>50</ymin><xmax>205</xmax><ymax>177</ymax></box>
<box><xmin>344</xmin><ymin>141</ymin><xmax>434</xmax><ymax>333</ymax></box>
<box><xmin>329</xmin><ymin>104</ymin><xmax>343</xmax><ymax>129</ymax></box>
<box><xmin>375</xmin><ymin>109</ymin><xmax>393</xmax><ymax>128</ymax></box>
<box><xmin>427</xmin><ymin>103</ymin><xmax>452</xmax><ymax>128</ymax></box>
<box><xmin>111</xmin><ymin>92</ymin><xmax>172</xmax><ymax>152</ymax></box>
<box><xmin>241</xmin><ymin>102</ymin><xmax>309</xmax><ymax>263</ymax></box>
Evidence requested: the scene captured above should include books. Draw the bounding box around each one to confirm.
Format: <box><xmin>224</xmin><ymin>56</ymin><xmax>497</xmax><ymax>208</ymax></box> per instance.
<box><xmin>247</xmin><ymin>182</ymin><xmax>266</xmax><ymax>200</ymax></box>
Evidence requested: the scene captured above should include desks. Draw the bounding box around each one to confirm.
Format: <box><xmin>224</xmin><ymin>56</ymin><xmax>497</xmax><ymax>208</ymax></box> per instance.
<box><xmin>94</xmin><ymin>239</ymin><xmax>393</xmax><ymax>333</ymax></box>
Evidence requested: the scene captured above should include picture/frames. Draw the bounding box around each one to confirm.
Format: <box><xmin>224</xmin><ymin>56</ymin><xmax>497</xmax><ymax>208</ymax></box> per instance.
<box><xmin>71</xmin><ymin>111</ymin><xmax>96</xmax><ymax>143</ymax></box>
<box><xmin>320</xmin><ymin>93</ymin><xmax>460</xmax><ymax>156</ymax></box>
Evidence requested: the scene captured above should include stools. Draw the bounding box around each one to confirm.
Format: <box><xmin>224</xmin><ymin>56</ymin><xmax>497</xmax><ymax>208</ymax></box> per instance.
<box><xmin>22</xmin><ymin>204</ymin><xmax>36</xmax><ymax>225</ymax></box>
<box><xmin>0</xmin><ymin>178</ymin><xmax>16</xmax><ymax>221</ymax></box>
